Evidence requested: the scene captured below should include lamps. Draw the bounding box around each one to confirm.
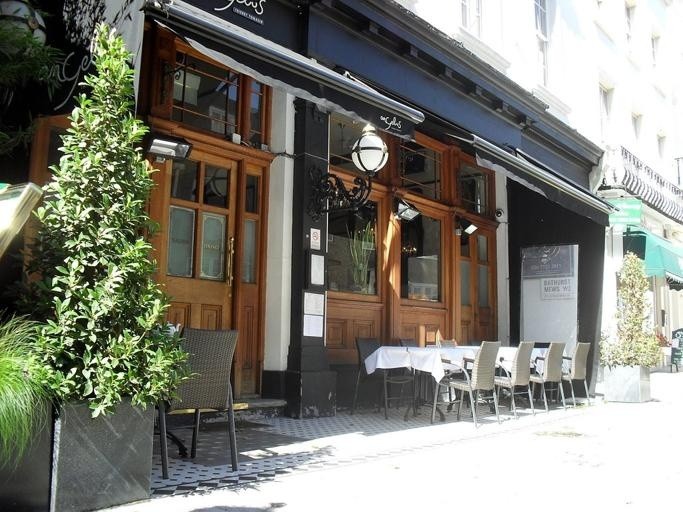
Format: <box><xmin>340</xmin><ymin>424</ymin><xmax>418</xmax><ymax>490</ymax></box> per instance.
<box><xmin>307</xmin><ymin>117</ymin><xmax>389</xmax><ymax>222</ymax></box>
<box><xmin>454</xmin><ymin>215</ymin><xmax>479</xmax><ymax>236</ymax></box>
<box><xmin>392</xmin><ymin>195</ymin><xmax>421</xmax><ymax>223</ymax></box>
<box><xmin>0</xmin><ymin>1</ymin><xmax>47</xmax><ymax>62</ymax></box>
<box><xmin>137</xmin><ymin>131</ymin><xmax>193</xmax><ymax>160</ymax></box>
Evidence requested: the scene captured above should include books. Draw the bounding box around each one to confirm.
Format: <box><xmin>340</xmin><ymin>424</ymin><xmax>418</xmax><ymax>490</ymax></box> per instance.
<box><xmin>439</xmin><ymin>339</ymin><xmax>456</xmax><ymax>348</ymax></box>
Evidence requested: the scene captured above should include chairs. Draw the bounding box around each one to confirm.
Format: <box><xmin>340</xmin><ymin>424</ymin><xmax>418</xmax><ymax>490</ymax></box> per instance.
<box><xmin>351</xmin><ymin>331</ymin><xmax>594</xmax><ymax>424</ymax></box>
<box><xmin>152</xmin><ymin>327</ymin><xmax>241</xmax><ymax>475</ymax></box>
